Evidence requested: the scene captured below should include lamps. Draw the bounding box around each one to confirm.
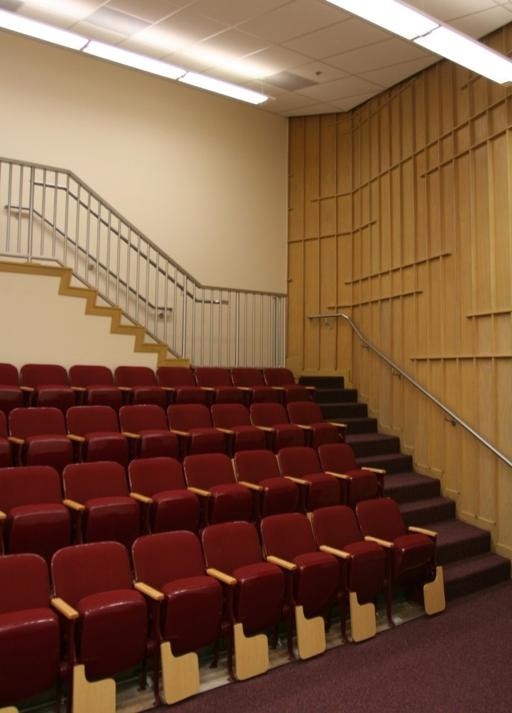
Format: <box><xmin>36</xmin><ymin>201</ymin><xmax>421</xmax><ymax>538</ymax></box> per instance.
<box><xmin>329</xmin><ymin>0</ymin><xmax>512</xmax><ymax>90</ymax></box>
<box><xmin>0</xmin><ymin>7</ymin><xmax>273</xmax><ymax>106</ymax></box>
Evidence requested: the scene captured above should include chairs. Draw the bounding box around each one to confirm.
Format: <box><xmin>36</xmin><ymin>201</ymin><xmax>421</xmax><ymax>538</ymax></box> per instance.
<box><xmin>2</xmin><ymin>466</ymin><xmax>87</xmax><ymax>550</ymax></box>
<box><xmin>52</xmin><ymin>541</ymin><xmax>163</xmax><ymax>710</ymax></box>
<box><xmin>70</xmin><ymin>363</ymin><xmax>131</xmax><ymax>410</ymax></box>
<box><xmin>259</xmin><ymin>513</ymin><xmax>353</xmax><ymax>660</ymax></box>
<box><xmin>234</xmin><ymin>449</ymin><xmax>311</xmax><ymax>510</ymax></box>
<box><xmin>18</xmin><ymin>361</ymin><xmax>85</xmax><ymax>411</ymax></box>
<box><xmin>288</xmin><ymin>403</ymin><xmax>347</xmax><ymax>444</ymax></box>
<box><xmin>132</xmin><ymin>530</ymin><xmax>239</xmax><ymax>706</ymax></box>
<box><xmin>117</xmin><ymin>404</ymin><xmax>190</xmax><ymax>465</ymax></box>
<box><xmin>354</xmin><ymin>497</ymin><xmax>445</xmax><ymax>616</ymax></box>
<box><xmin>155</xmin><ymin>366</ymin><xmax>215</xmax><ymax>404</ymax></box>
<box><xmin>249</xmin><ymin>405</ymin><xmax>310</xmax><ymax>448</ymax></box>
<box><xmin>2</xmin><ymin>553</ymin><xmax>81</xmax><ymax>710</ymax></box>
<box><xmin>212</xmin><ymin>403</ymin><xmax>275</xmax><ymax>450</ymax></box>
<box><xmin>167</xmin><ymin>404</ymin><xmax>234</xmax><ymax>457</ymax></box>
<box><xmin>230</xmin><ymin>366</ymin><xmax>286</xmax><ymax>403</ymax></box>
<box><xmin>194</xmin><ymin>366</ymin><xmax>252</xmax><ymax>404</ymax></box>
<box><xmin>200</xmin><ymin>520</ymin><xmax>298</xmax><ymax>683</ymax></box>
<box><xmin>2</xmin><ymin>361</ymin><xmax>19</xmax><ymax>414</ymax></box>
<box><xmin>278</xmin><ymin>446</ymin><xmax>351</xmax><ymax>508</ymax></box>
<box><xmin>319</xmin><ymin>444</ymin><xmax>385</xmax><ymax>503</ymax></box>
<box><xmin>9</xmin><ymin>406</ymin><xmax>86</xmax><ymax>466</ymax></box>
<box><xmin>65</xmin><ymin>404</ymin><xmax>141</xmax><ymax>465</ymax></box>
<box><xmin>184</xmin><ymin>453</ymin><xmax>263</xmax><ymax>518</ymax></box>
<box><xmin>63</xmin><ymin>460</ymin><xmax>155</xmax><ymax>542</ymax></box>
<box><xmin>129</xmin><ymin>456</ymin><xmax>212</xmax><ymax>528</ymax></box>
<box><xmin>114</xmin><ymin>365</ymin><xmax>175</xmax><ymax>406</ymax></box>
<box><xmin>264</xmin><ymin>367</ymin><xmax>316</xmax><ymax>402</ymax></box>
<box><xmin>1</xmin><ymin>410</ymin><xmax>25</xmax><ymax>464</ymax></box>
<box><xmin>306</xmin><ymin>505</ymin><xmax>394</xmax><ymax>645</ymax></box>
